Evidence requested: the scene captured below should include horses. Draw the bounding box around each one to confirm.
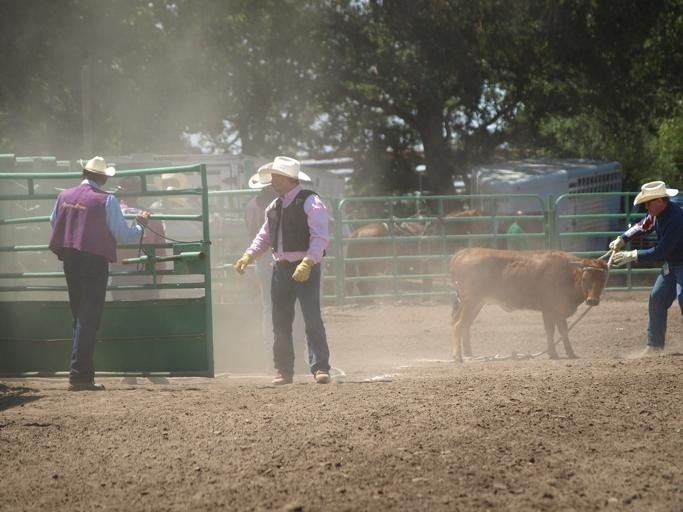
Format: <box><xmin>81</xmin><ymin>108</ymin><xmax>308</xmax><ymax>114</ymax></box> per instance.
<box><xmin>344</xmin><ymin>207</ymin><xmax>487</xmax><ymax>296</ymax></box>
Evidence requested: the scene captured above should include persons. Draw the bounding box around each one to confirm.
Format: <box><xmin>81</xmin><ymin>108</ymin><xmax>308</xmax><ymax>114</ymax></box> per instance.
<box><xmin>46</xmin><ymin>153</ymin><xmax>153</xmax><ymax>394</ymax></box>
<box><xmin>229</xmin><ymin>154</ymin><xmax>336</xmax><ymax>387</ymax></box>
<box><xmin>239</xmin><ymin>161</ymin><xmax>314</xmax><ymax>375</ymax></box>
<box><xmin>607</xmin><ymin>179</ymin><xmax>681</xmax><ymax>360</ymax></box>
<box><xmin>0</xmin><ymin>154</ymin><xmax>532</xmax><ymax>348</ymax></box>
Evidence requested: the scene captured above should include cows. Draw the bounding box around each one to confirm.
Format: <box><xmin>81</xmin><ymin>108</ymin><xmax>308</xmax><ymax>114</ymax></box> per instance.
<box><xmin>449</xmin><ymin>247</ymin><xmax>608</xmax><ymax>363</ymax></box>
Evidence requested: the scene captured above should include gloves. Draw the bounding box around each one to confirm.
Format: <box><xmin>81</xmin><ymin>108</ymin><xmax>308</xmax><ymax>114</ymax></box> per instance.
<box><xmin>292</xmin><ymin>257</ymin><xmax>314</xmax><ymax>282</ymax></box>
<box><xmin>609</xmin><ymin>236</ymin><xmax>624</xmax><ymax>251</ymax></box>
<box><xmin>612</xmin><ymin>250</ymin><xmax>638</xmax><ymax>266</ymax></box>
<box><xmin>235</xmin><ymin>252</ymin><xmax>253</xmax><ymax>274</ymax></box>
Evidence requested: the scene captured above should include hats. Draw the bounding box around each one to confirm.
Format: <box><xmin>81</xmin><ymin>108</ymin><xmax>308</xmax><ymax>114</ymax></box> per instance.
<box><xmin>79</xmin><ymin>155</ymin><xmax>116</xmax><ymax>177</ymax></box>
<box><xmin>272</xmin><ymin>156</ymin><xmax>312</xmax><ymax>182</ymax></box>
<box><xmin>634</xmin><ymin>180</ymin><xmax>679</xmax><ymax>204</ymax></box>
<box><xmin>248</xmin><ymin>155</ymin><xmax>273</xmax><ymax>189</ymax></box>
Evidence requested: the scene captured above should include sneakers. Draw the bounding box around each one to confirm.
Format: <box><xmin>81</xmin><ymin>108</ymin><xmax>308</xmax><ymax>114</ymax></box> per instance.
<box><xmin>67</xmin><ymin>383</ymin><xmax>105</xmax><ymax>390</ymax></box>
<box><xmin>314</xmin><ymin>370</ymin><xmax>330</xmax><ymax>383</ymax></box>
<box><xmin>272</xmin><ymin>376</ymin><xmax>293</xmax><ymax>385</ymax></box>
